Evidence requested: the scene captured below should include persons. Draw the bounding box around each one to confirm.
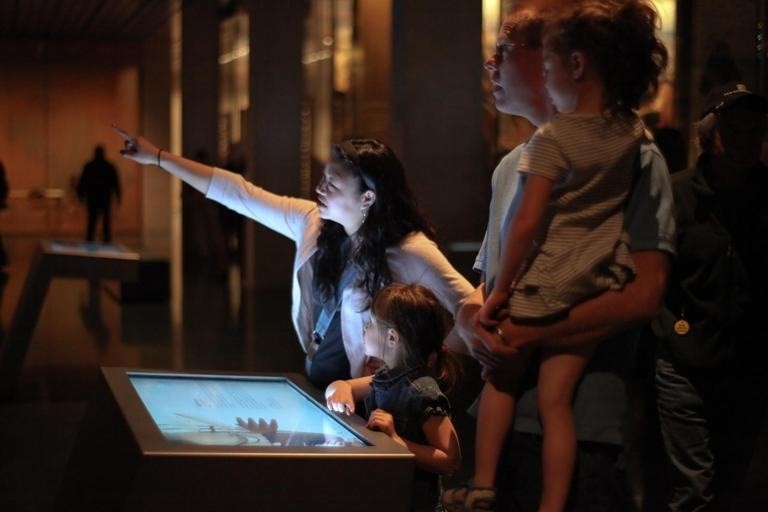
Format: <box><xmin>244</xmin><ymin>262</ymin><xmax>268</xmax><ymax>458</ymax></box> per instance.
<box><xmin>78</xmin><ymin>143</ymin><xmax>123</xmax><ymax>243</ymax></box>
<box><xmin>649</xmin><ymin>81</ymin><xmax>768</xmax><ymax>512</ymax></box>
<box><xmin>110</xmin><ymin>123</ymin><xmax>475</xmax><ymax>418</ymax></box>
<box><xmin>324</xmin><ymin>280</ymin><xmax>459</xmax><ymax>512</ymax></box>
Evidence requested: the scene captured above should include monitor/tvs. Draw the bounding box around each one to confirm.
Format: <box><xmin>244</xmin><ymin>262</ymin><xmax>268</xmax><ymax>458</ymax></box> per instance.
<box><xmin>100</xmin><ymin>362</ymin><xmax>417</xmax><ymax>458</ymax></box>
<box><xmin>42</xmin><ymin>240</ymin><xmax>141</xmax><ymax>260</ymax></box>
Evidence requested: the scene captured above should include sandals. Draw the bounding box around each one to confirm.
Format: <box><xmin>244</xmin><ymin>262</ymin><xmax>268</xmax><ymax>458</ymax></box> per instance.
<box><xmin>439</xmin><ymin>487</ymin><xmax>499</xmax><ymax>512</ymax></box>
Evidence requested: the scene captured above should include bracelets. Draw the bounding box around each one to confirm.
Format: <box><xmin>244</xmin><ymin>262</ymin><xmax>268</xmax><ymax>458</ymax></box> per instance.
<box><xmin>159</xmin><ymin>149</ymin><xmax>162</xmax><ymax>168</ymax></box>
<box><xmin>493</xmin><ymin>322</ymin><xmax>510</xmax><ymax>346</ymax></box>
